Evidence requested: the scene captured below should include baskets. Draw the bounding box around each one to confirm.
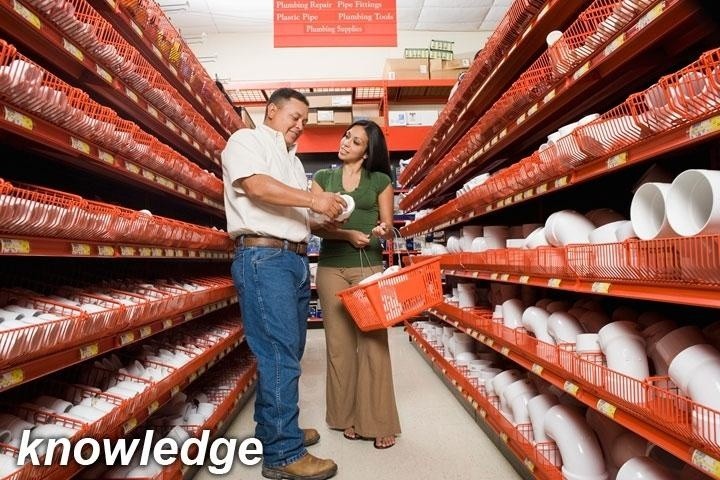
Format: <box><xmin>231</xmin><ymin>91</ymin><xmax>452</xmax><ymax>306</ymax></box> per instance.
<box><xmin>334</xmin><ymin>227</ymin><xmax>445</xmax><ymax>333</ymax></box>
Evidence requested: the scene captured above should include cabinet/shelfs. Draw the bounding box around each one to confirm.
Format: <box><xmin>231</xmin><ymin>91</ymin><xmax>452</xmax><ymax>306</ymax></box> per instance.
<box><xmin>214</xmin><ymin>78</ymin><xmax>467</xmax><ymax>325</ymax></box>
<box><xmin>375</xmin><ymin>0</ymin><xmax>720</xmax><ymax>480</ymax></box>
<box><xmin>0</xmin><ymin>0</ymin><xmax>256</xmax><ymax>479</ymax></box>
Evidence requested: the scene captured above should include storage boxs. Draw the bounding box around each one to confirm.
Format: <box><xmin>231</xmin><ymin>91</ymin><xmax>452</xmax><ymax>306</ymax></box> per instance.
<box><xmin>306</xmin><ymin>93</ymin><xmax>385</xmax><ymax>129</ymax></box>
<box><xmin>387</xmin><ymin>110</ymin><xmax>440</xmax><ymax>127</ymax></box>
<box><xmin>383</xmin><ymin>40</ymin><xmax>473</xmax><ymax>81</ymax></box>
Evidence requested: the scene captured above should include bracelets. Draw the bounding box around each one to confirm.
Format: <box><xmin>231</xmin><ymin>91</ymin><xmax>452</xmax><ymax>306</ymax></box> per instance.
<box><xmin>310</xmin><ymin>189</ymin><xmax>317</xmax><ymax>209</ymax></box>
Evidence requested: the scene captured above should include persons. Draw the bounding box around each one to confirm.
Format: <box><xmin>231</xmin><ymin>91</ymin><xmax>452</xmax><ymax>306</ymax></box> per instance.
<box><xmin>307</xmin><ymin>119</ymin><xmax>402</xmax><ymax>450</ymax></box>
<box><xmin>220</xmin><ymin>87</ymin><xmax>349</xmax><ymax>480</ymax></box>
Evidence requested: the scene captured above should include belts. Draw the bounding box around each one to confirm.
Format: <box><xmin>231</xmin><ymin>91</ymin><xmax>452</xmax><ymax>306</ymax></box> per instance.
<box><xmin>234</xmin><ymin>233</ymin><xmax>310</xmax><ymax>257</ymax></box>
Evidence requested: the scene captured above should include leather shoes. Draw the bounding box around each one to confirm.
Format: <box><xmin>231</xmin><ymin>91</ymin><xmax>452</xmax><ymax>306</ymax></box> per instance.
<box><xmin>260</xmin><ymin>451</ymin><xmax>338</xmax><ymax>477</ymax></box>
<box><xmin>257</xmin><ymin>429</ymin><xmax>320</xmax><ymax>446</ymax></box>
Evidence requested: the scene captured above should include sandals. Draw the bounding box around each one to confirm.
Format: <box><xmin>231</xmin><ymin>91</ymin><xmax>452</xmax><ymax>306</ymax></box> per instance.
<box><xmin>343</xmin><ymin>426</ymin><xmax>397</xmax><ymax>448</ymax></box>
